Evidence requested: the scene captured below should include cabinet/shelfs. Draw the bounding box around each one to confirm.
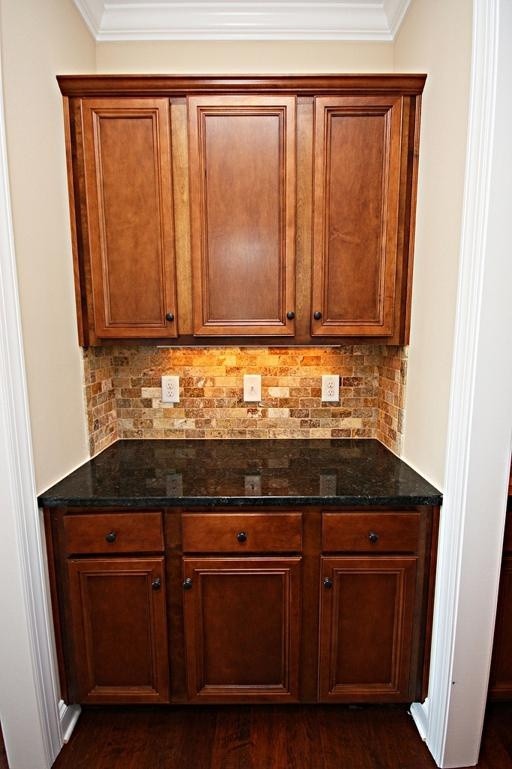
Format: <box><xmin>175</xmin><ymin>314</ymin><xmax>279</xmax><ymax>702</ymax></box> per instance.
<box><xmin>43</xmin><ymin>507</ymin><xmax>309</xmax><ymax>705</ymax></box>
<box><xmin>54</xmin><ymin>74</ymin><xmax>191</xmax><ymax>348</ymax></box>
<box><xmin>184</xmin><ymin>74</ymin><xmax>428</xmax><ymax>348</ymax></box>
<box><xmin>313</xmin><ymin>506</ymin><xmax>440</xmax><ymax>705</ymax></box>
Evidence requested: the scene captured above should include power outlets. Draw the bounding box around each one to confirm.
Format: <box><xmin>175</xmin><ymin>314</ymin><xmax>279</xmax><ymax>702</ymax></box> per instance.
<box><xmin>320</xmin><ymin>375</ymin><xmax>341</xmax><ymax>401</ymax></box>
<box><xmin>160</xmin><ymin>374</ymin><xmax>180</xmax><ymax>402</ymax></box>
